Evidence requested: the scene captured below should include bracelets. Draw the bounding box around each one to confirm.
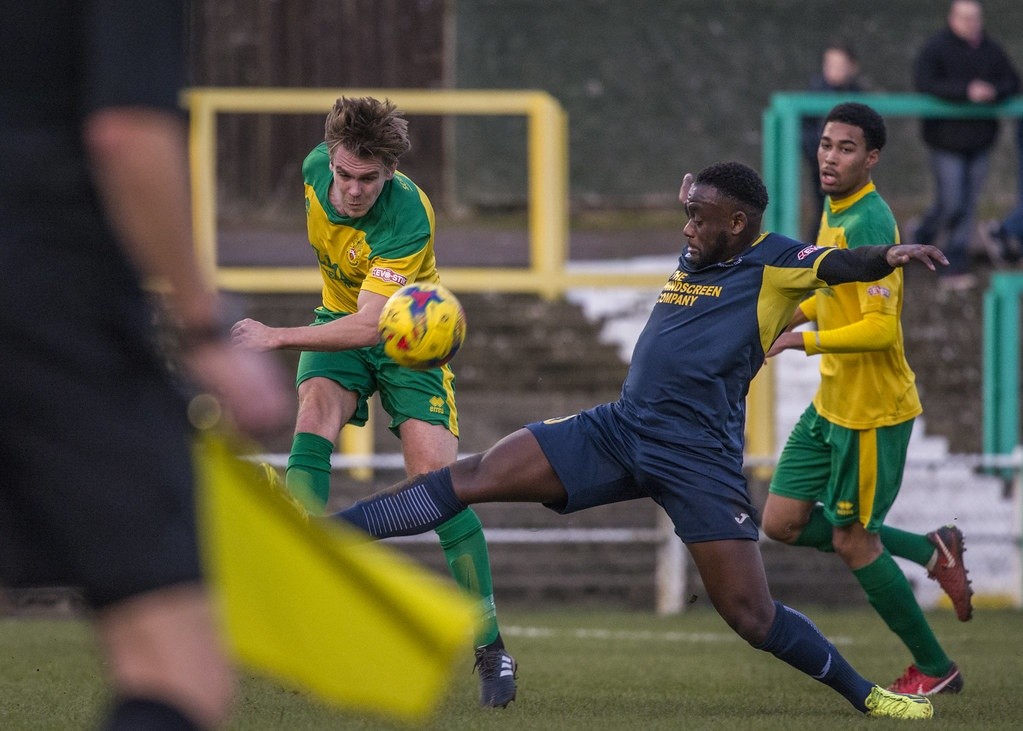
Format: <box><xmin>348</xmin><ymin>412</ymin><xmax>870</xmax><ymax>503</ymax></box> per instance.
<box><xmin>173</xmin><ymin>318</ymin><xmax>236</xmax><ymax>349</ymax></box>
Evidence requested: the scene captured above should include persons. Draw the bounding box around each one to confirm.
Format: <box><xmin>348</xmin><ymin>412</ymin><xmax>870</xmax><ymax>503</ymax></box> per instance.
<box><xmin>761</xmin><ymin>103</ymin><xmax>975</xmax><ymax>699</ymax></box>
<box><xmin>800</xmin><ymin>37</ymin><xmax>887</xmax><ymax>245</ymax></box>
<box><xmin>979</xmin><ymin>113</ymin><xmax>1023</xmax><ymax>267</ymax></box>
<box><xmin>0</xmin><ymin>1</ymin><xmax>304</xmax><ymax>730</ymax></box>
<box><xmin>308</xmin><ymin>163</ymin><xmax>950</xmax><ymax>722</ymax></box>
<box><xmin>897</xmin><ymin>0</ymin><xmax>1023</xmax><ymax>263</ymax></box>
<box><xmin>229</xmin><ymin>94</ymin><xmax>519</xmax><ymax>710</ymax></box>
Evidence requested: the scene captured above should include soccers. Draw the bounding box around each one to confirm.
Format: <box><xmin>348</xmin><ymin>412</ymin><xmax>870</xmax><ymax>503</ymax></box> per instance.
<box><xmin>378</xmin><ymin>282</ymin><xmax>467</xmax><ymax>371</ymax></box>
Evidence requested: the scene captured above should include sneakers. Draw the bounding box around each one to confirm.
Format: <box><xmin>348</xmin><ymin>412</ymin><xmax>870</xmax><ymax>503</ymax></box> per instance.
<box><xmin>863</xmin><ymin>685</ymin><xmax>934</xmax><ymax>719</ymax></box>
<box><xmin>925</xmin><ymin>525</ymin><xmax>974</xmax><ymax>621</ymax></box>
<box><xmin>886</xmin><ymin>662</ymin><xmax>964</xmax><ymax>697</ymax></box>
<box><xmin>472</xmin><ymin>648</ymin><xmax>519</xmax><ymax>707</ymax></box>
